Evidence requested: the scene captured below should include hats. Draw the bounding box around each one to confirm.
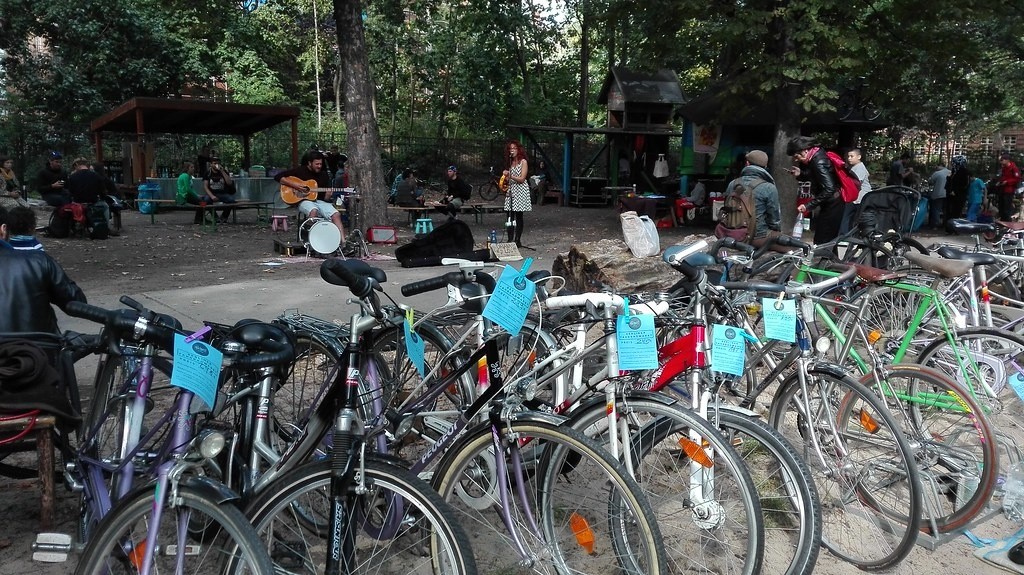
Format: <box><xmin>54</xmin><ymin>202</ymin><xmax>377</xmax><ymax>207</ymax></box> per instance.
<box><xmin>745</xmin><ymin>150</ymin><xmax>768</xmax><ymax>167</ymax></box>
<box><xmin>786</xmin><ymin>139</ymin><xmax>813</xmax><ymax>155</ymax></box>
<box><xmin>447</xmin><ymin>166</ymin><xmax>457</xmax><ymax>172</ymax></box>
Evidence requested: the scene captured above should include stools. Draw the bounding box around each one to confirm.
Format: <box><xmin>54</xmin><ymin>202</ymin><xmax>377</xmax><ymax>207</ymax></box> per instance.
<box><xmin>297</xmin><ymin>212</ymin><xmax>309</xmax><ymax>239</ymax></box>
<box><xmin>416</xmin><ymin>219</ymin><xmax>433</xmax><ymax>233</ymax></box>
<box><xmin>0</xmin><ymin>416</ymin><xmax>55</xmax><ymax>529</ymax></box>
<box><xmin>272</xmin><ymin>216</ymin><xmax>286</xmax><ymax>232</ymax></box>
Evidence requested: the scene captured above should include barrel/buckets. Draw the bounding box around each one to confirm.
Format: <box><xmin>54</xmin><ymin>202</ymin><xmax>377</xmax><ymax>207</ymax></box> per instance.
<box><xmin>138</xmin><ymin>183</ymin><xmax>159</xmax><ymax>213</ymax></box>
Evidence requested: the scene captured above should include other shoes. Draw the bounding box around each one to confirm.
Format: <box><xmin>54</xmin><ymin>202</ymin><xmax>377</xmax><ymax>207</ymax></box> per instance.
<box><xmin>343</xmin><ymin>246</ymin><xmax>358</xmax><ymax>257</ymax></box>
<box><xmin>515</xmin><ymin>241</ymin><xmax>521</xmax><ymax>248</ymax></box>
<box><xmin>679</xmin><ymin>222</ymin><xmax>686</xmax><ymax>227</ymax></box>
<box><xmin>193</xmin><ymin>216</ymin><xmax>228</xmax><ymax>225</ymax></box>
<box><xmin>925</xmin><ymin>223</ymin><xmax>960</xmax><ymax>236</ymax></box>
<box><xmin>413</xmin><ymin>222</ymin><xmax>424</xmax><ymax>228</ymax></box>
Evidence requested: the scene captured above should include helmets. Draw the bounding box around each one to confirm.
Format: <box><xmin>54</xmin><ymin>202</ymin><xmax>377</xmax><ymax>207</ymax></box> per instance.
<box><xmin>952</xmin><ymin>156</ymin><xmax>967</xmax><ymax>168</ymax></box>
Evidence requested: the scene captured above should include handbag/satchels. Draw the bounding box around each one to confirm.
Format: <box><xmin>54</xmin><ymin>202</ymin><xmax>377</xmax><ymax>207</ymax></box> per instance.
<box><xmin>6</xmin><ymin>179</ymin><xmax>16</xmax><ymax>192</ymax></box>
<box><xmin>653</xmin><ymin>157</ymin><xmax>669</xmax><ymax>178</ymax></box>
<box><xmin>620</xmin><ymin>210</ymin><xmax>661</xmax><ymax>259</ymax></box>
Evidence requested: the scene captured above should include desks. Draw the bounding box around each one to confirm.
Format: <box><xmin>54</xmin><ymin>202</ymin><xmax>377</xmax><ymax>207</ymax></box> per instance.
<box><xmin>618</xmin><ymin>197</ymin><xmax>678</xmax><ymax>230</ymax></box>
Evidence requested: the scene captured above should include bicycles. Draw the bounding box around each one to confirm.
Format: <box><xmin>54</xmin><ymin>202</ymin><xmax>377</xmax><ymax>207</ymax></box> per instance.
<box><xmin>479</xmin><ymin>167</ymin><xmax>504</xmax><ymax>201</ymax></box>
<box><xmin>25</xmin><ymin>208</ymin><xmax>1024</xmax><ymax>575</ymax></box>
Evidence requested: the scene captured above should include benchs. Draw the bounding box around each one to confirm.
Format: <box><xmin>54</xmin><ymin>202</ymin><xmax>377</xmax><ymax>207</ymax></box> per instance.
<box><xmin>137</xmin><ymin>197</ymin><xmax>274</xmax><ymax>230</ymax></box>
<box><xmin>390</xmin><ymin>203</ymin><xmax>506</xmax><ymax>224</ymax></box>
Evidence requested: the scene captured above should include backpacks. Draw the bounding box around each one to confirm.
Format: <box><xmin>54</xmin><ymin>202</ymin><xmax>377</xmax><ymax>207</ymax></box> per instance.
<box><xmin>714</xmin><ymin>176</ymin><xmax>768</xmax><ymax>244</ymax></box>
<box><xmin>811</xmin><ymin>150</ymin><xmax>862</xmax><ymax>203</ymax></box>
<box><xmin>462</xmin><ymin>184</ymin><xmax>472</xmax><ymax>202</ymax></box>
<box><xmin>45</xmin><ymin>200</ymin><xmax>112</xmax><ymax>240</ymax></box>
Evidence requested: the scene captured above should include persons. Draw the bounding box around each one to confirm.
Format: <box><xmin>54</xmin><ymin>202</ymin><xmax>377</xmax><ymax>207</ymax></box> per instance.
<box><xmin>503</xmin><ymin>140</ymin><xmax>532</xmax><ymax>249</ymax></box>
<box><xmin>0</xmin><ymin>156</ymin><xmax>31</xmax><ymax>213</ymax></box>
<box><xmin>8</xmin><ymin>206</ymin><xmax>44</xmax><ymax>251</ymax></box>
<box><xmin>620</xmin><ymin>153</ymin><xmax>705</xmax><ymax>228</ymax></box>
<box><xmin>437</xmin><ymin>166</ymin><xmax>470</xmax><ymax>221</ymax></box>
<box><xmin>0</xmin><ymin>204</ymin><xmax>87</xmax><ymax>458</ymax></box>
<box><xmin>301</xmin><ymin>145</ymin><xmax>349</xmax><ymax>202</ymax></box>
<box><xmin>275</xmin><ymin>152</ymin><xmax>346</xmax><ymax>250</ymax></box>
<box><xmin>177</xmin><ymin>162</ymin><xmax>212</xmax><ymax>225</ymax></box>
<box><xmin>204</xmin><ymin>156</ymin><xmax>236</xmax><ymax>222</ymax></box>
<box><xmin>531</xmin><ymin>162</ymin><xmax>548</xmax><ymax>205</ymax></box>
<box><xmin>784</xmin><ymin>139</ymin><xmax>873</xmax><ymax>249</ymax></box>
<box><xmin>391</xmin><ymin>167</ymin><xmax>425</xmax><ymax>229</ymax></box>
<box><xmin>198</xmin><ymin>146</ymin><xmax>212</xmax><ymax>178</ymax></box>
<box><xmin>722</xmin><ymin>150</ymin><xmax>806</xmax><ymax>264</ymax></box>
<box><xmin>35</xmin><ymin>151</ymin><xmax>121</xmax><ymax>238</ymax></box>
<box><xmin>886</xmin><ymin>150</ymin><xmax>1024</xmax><ymax>235</ymax></box>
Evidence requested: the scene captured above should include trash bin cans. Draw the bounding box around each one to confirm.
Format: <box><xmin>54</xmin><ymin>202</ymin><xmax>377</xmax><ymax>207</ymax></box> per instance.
<box><xmin>138</xmin><ymin>183</ymin><xmax>161</xmax><ymax>215</ymax></box>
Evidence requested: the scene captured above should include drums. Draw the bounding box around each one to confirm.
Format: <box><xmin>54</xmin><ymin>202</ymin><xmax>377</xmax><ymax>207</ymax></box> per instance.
<box><xmin>298</xmin><ymin>216</ymin><xmax>343</xmax><ymax>256</ymax></box>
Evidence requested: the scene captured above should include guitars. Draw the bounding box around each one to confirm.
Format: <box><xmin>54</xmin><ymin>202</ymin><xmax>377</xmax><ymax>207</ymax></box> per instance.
<box><xmin>279</xmin><ymin>175</ymin><xmax>356</xmax><ymax>205</ymax></box>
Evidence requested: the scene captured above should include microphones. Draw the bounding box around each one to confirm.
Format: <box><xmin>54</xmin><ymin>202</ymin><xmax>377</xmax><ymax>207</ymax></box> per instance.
<box><xmin>509</xmin><ymin>151</ymin><xmax>514</xmax><ymax>158</ymax></box>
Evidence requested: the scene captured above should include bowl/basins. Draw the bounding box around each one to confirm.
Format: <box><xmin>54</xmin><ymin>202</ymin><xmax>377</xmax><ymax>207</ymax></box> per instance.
<box><xmin>199</xmin><ymin>202</ymin><xmax>208</xmax><ymax>206</ymax></box>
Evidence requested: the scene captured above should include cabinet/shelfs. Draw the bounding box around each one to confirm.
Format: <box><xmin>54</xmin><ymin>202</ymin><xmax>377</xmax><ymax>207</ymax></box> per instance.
<box><xmin>569</xmin><ymin>176</ymin><xmax>611</xmax><ymax>206</ymax></box>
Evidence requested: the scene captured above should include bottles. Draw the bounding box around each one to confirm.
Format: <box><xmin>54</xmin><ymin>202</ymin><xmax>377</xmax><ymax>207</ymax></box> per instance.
<box><xmin>491</xmin><ymin>227</ymin><xmax>497</xmax><ymax>243</ymax></box>
<box><xmin>792</xmin><ymin>210</ymin><xmax>804</xmax><ymax>242</ymax></box>
<box><xmin>633</xmin><ymin>184</ymin><xmax>637</xmax><ymax>194</ymax></box>
<box><xmin>625</xmin><ymin>190</ymin><xmax>634</xmax><ymax>198</ymax></box>
<box><xmin>151</xmin><ymin>167</ymin><xmax>175</xmax><ymax>179</ymax></box>
